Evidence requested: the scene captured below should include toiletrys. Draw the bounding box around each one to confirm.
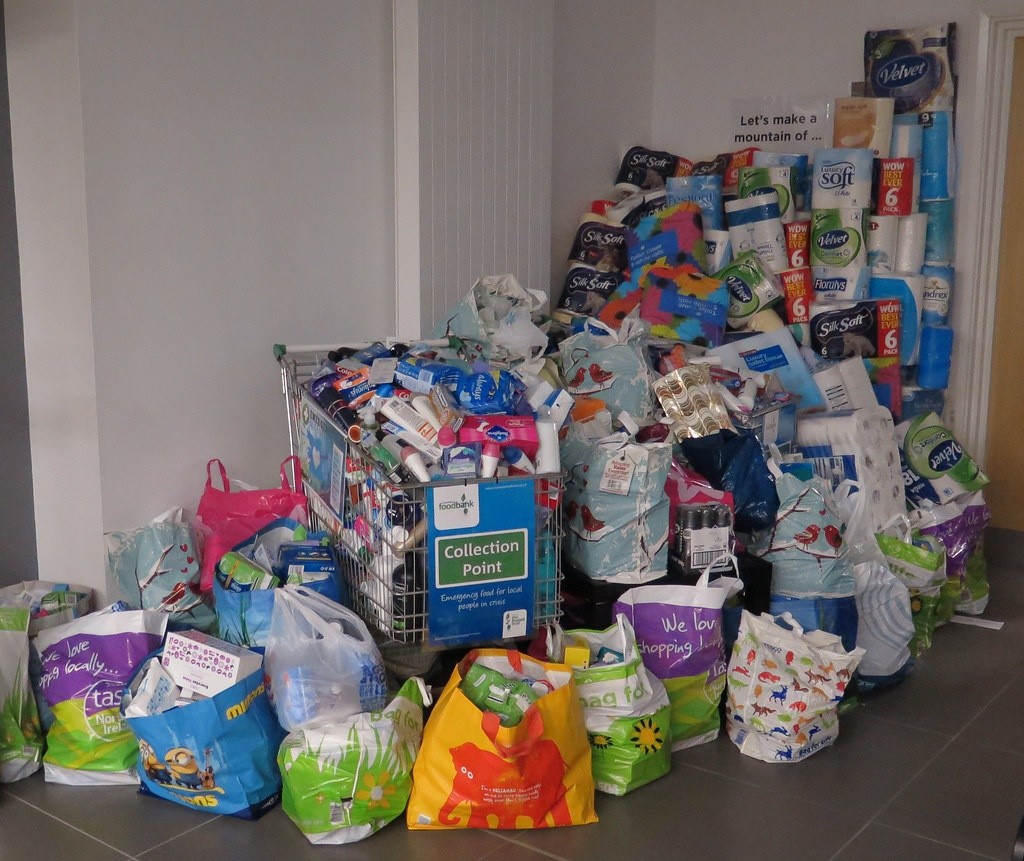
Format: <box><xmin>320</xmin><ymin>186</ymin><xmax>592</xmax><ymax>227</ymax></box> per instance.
<box><xmin>373</xmin><ymin>380</ymin><xmax>561</xmax><ymax>482</ymax></box>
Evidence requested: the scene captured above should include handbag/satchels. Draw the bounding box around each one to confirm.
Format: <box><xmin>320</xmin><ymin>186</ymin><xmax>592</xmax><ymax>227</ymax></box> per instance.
<box><xmin>118</xmin><ymin>629</ymin><xmax>283</xmax><ymax>821</ymax></box>
<box><xmin>194</xmin><ymin>458</ymin><xmax>310</xmax><ymax>597</ymax></box>
<box><xmin>35</xmin><ymin>599</ymin><xmax>169</xmax><ymax>787</ymax></box>
<box><xmin>101</xmin><ymin>505</ymin><xmax>218</xmax><ymax>631</ymax></box>
<box><xmin>209</xmin><ymin>517</ymin><xmax>350</xmax><ymax>659</ymax></box>
<box><xmin>406</xmin><ymin>267</ymin><xmax>989</xmax><ymax>830</ymax></box>
<box><xmin>264</xmin><ymin>584</ymin><xmax>388</xmax><ymax>734</ymax></box>
<box><xmin>0</xmin><ymin>579</ymin><xmax>94</xmax><ymax>786</ymax></box>
<box><xmin>278</xmin><ymin>676</ymin><xmax>433</xmax><ymax>847</ymax></box>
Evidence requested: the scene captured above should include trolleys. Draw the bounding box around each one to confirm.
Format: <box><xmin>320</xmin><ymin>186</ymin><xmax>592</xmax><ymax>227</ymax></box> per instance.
<box><xmin>271</xmin><ymin>322</ymin><xmax>572</xmax><ymax>646</ymax></box>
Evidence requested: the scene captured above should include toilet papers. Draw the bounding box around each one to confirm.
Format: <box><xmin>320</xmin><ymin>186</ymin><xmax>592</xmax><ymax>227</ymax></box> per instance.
<box><xmin>550</xmin><ymin>18</ymin><xmax>993</xmax><ymax>575</ymax></box>
